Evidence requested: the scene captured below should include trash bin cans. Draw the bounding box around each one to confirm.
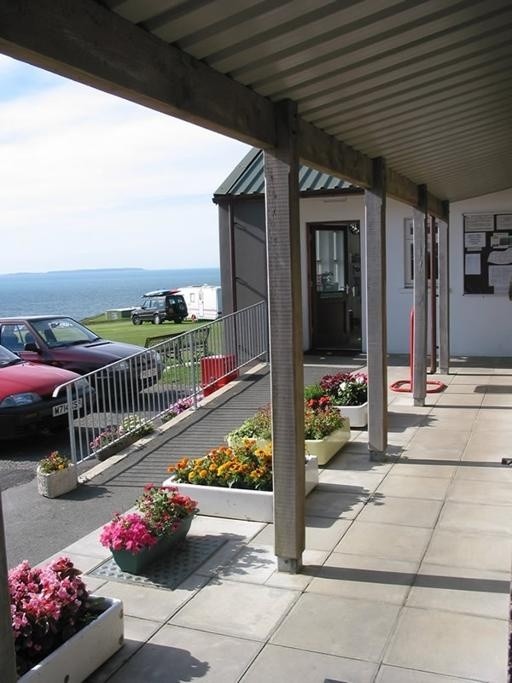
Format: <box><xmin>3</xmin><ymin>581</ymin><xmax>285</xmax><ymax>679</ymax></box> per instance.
<box><xmin>200</xmin><ymin>354</ymin><xmax>239</xmax><ymax>397</ymax></box>
<box><xmin>344</xmin><ymin>309</ymin><xmax>353</xmax><ymax>344</ymax></box>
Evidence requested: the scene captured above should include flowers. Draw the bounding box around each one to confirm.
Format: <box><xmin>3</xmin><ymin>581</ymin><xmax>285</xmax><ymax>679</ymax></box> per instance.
<box><xmin>159</xmin><ymin>396</ymin><xmax>206</xmax><ymax>424</ymax></box>
<box><xmin>118</xmin><ymin>414</ymin><xmax>154</xmax><ymax>435</ymax></box>
<box><xmin>131</xmin><ymin>481</ymin><xmax>205</xmax><ymax>536</ymax></box>
<box><xmin>314</xmin><ymin>371</ymin><xmax>369</xmax><ymax>406</ymax></box>
<box><xmin>230</xmin><ymin>394</ymin><xmax>344</xmax><ymax>442</ymax></box>
<box><xmin>88</xmin><ymin>430</ymin><xmax>126</xmax><ymax>449</ymax></box>
<box><xmin>37</xmin><ymin>451</ymin><xmax>69</xmax><ymax>472</ymax></box>
<box><xmin>169</xmin><ymin>436</ymin><xmax>275</xmax><ymax>488</ymax></box>
<box><xmin>99</xmin><ymin>511</ymin><xmax>161</xmax><ymax>555</ymax></box>
<box><xmin>5</xmin><ymin>557</ymin><xmax>106</xmax><ymax>665</ymax></box>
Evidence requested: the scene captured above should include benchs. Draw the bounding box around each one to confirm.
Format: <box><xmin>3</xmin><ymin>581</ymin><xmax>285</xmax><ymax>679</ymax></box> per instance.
<box><xmin>144</xmin><ymin>328</ymin><xmax>210</xmax><ymax>367</ymax></box>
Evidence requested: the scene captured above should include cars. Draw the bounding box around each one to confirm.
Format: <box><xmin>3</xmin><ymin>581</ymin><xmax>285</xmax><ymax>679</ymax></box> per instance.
<box><xmin>0</xmin><ymin>341</ymin><xmax>97</xmax><ymax>451</ymax></box>
<box><xmin>0</xmin><ymin>314</ymin><xmax>166</xmax><ymax>406</ymax></box>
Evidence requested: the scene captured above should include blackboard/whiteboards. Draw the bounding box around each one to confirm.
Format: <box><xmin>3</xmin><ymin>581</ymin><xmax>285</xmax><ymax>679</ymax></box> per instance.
<box><xmin>461</xmin><ymin>212</ymin><xmax>512</xmax><ymax>297</ymax></box>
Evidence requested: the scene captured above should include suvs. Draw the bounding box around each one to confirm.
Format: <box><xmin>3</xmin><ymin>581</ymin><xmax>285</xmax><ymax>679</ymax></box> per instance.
<box><xmin>130</xmin><ymin>289</ymin><xmax>188</xmax><ymax>326</ymax></box>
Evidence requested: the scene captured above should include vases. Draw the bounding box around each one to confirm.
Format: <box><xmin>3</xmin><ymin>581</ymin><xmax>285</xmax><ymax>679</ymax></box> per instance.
<box><xmin>92</xmin><ymin>449</ymin><xmax>123</xmax><ymax>462</ymax></box>
<box><xmin>225</xmin><ymin>418</ymin><xmax>350</xmax><ymax>466</ymax></box>
<box><xmin>330</xmin><ymin>403</ymin><xmax>369</xmax><ymax>428</ymax></box>
<box><xmin>33</xmin><ymin>463</ymin><xmax>78</xmax><ymax>498</ymax></box>
<box><xmin>108</xmin><ymin>537</ymin><xmax>164</xmax><ymax>575</ymax></box>
<box><xmin>127</xmin><ymin>436</ymin><xmax>144</xmax><ymax>445</ymax></box>
<box><xmin>153</xmin><ymin>509</ymin><xmax>197</xmax><ymax>546</ymax></box>
<box><xmin>161</xmin><ymin>450</ymin><xmax>322</xmax><ymax>523</ymax></box>
<box><xmin>15</xmin><ymin>596</ymin><xmax>124</xmax><ymax>682</ymax></box>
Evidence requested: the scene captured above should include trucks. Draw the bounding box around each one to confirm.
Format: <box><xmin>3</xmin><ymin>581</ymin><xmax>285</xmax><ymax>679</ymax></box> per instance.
<box><xmin>171</xmin><ymin>284</ymin><xmax>225</xmax><ymax>324</ymax></box>
